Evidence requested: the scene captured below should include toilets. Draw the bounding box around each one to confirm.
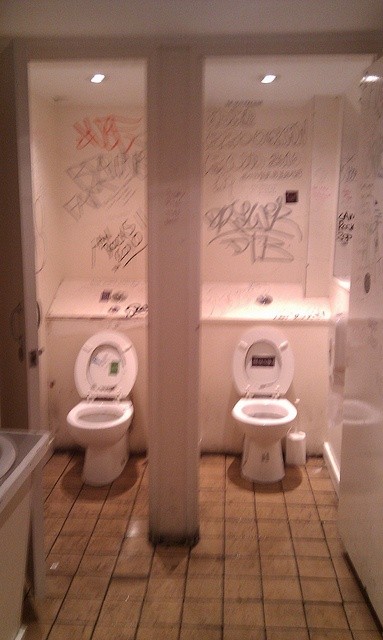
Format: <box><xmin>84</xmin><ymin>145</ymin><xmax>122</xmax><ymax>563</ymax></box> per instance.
<box><xmin>230</xmin><ymin>325</ymin><xmax>298</xmax><ymax>483</ymax></box>
<box><xmin>66</xmin><ymin>330</ymin><xmax>139</xmax><ymax>489</ymax></box>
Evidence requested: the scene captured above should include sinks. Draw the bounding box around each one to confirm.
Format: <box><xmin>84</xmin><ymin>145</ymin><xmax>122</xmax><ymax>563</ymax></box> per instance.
<box><xmin>0</xmin><ymin>435</ymin><xmax>17</xmax><ymax>479</ymax></box>
